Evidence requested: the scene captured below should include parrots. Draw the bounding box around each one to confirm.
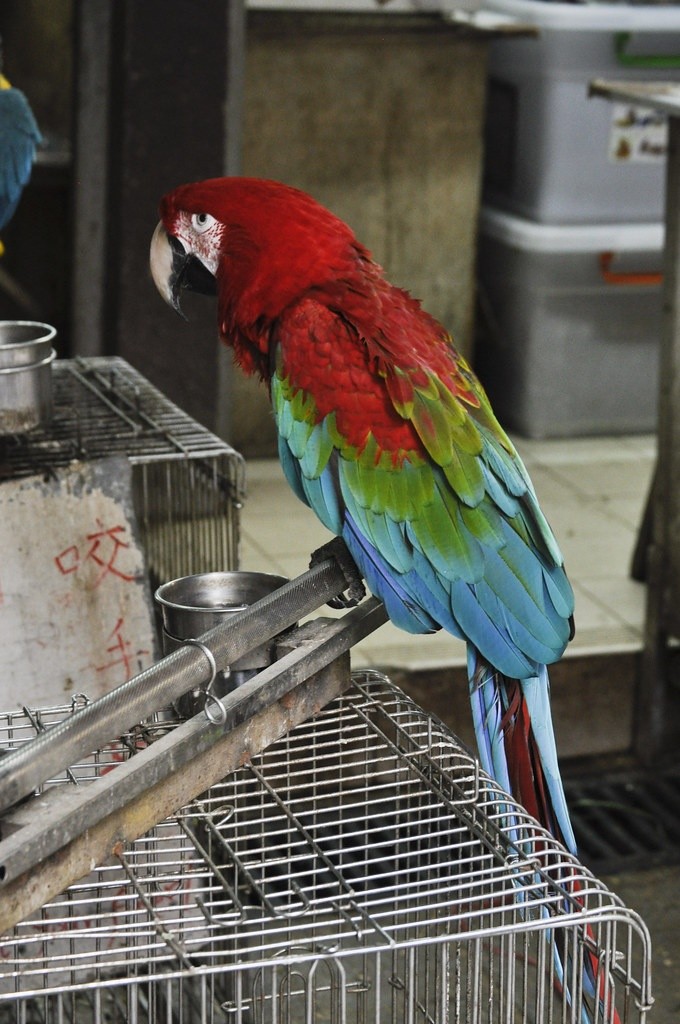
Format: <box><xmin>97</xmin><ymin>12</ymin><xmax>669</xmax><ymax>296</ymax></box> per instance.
<box><xmin>0</xmin><ymin>73</ymin><xmax>42</xmax><ymax>251</ymax></box>
<box><xmin>149</xmin><ymin>177</ymin><xmax>629</xmax><ymax>1024</ymax></box>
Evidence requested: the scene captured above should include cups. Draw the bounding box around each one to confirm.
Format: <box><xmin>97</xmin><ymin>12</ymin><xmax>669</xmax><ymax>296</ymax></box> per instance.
<box><xmin>155</xmin><ymin>572</ymin><xmax>298</xmax><ymax>718</ymax></box>
<box><xmin>0</xmin><ymin>322</ymin><xmax>57</xmax><ymax>437</ymax></box>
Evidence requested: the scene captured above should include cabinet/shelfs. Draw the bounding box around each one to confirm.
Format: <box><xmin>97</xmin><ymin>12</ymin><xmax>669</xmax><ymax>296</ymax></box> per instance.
<box><xmin>222</xmin><ymin>2</ymin><xmax>542</xmax><ymax>458</ymax></box>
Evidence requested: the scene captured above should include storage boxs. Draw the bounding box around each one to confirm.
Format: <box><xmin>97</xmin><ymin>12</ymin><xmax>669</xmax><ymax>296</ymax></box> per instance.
<box><xmin>449</xmin><ymin>0</ymin><xmax>680</xmax><ymax>439</ymax></box>
